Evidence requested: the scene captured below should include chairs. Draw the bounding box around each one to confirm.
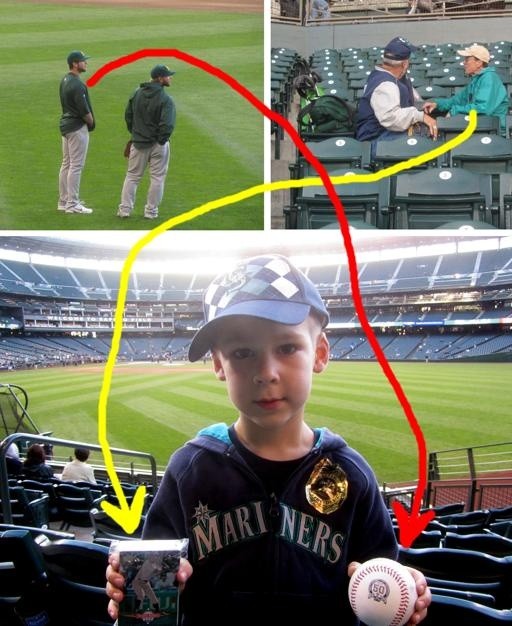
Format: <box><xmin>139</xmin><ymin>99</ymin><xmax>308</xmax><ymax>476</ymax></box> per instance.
<box><xmin>380</xmin><ymin>497</ymin><xmax>511</xmax><ymax>625</ymax></box>
<box><xmin>1</xmin><ymin>258</ymin><xmax>230</xmax><ymax>369</ymax></box>
<box><xmin>0</xmin><ymin>443</ymin><xmax>154</xmax><ymax>625</ymax></box>
<box><xmin>299</xmin><ymin>246</ymin><xmax>511</xmax><ymax>362</ymax></box>
<box><xmin>271</xmin><ymin>35</ymin><xmax>512</xmax><ymax>229</ymax></box>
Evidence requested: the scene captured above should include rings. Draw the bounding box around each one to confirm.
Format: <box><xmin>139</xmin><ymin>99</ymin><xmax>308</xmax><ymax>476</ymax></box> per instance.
<box><xmin>433</xmin><ymin>126</ymin><xmax>437</xmax><ymax>128</ymax></box>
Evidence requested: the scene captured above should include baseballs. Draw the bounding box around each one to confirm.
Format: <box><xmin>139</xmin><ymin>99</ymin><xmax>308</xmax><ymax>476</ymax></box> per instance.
<box><xmin>348</xmin><ymin>556</ymin><xmax>418</xmax><ymax>626</ymax></box>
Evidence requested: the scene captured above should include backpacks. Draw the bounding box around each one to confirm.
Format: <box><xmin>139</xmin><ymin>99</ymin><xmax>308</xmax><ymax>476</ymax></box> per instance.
<box><xmin>297</xmin><ymin>93</ymin><xmax>357</xmax><ymax>133</ymax></box>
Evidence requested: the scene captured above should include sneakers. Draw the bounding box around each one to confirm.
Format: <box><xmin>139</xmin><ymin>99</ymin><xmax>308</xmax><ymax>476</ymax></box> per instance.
<box><xmin>58</xmin><ymin>205</ymin><xmax>92</xmax><ymax>214</ymax></box>
<box><xmin>145</xmin><ymin>213</ymin><xmax>159</xmax><ymax>219</ymax></box>
<box><xmin>116</xmin><ymin>211</ymin><xmax>130</xmax><ymax>217</ymax></box>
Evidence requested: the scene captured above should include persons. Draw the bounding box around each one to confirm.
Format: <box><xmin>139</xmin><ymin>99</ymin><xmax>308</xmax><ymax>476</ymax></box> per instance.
<box><xmin>422</xmin><ymin>44</ymin><xmax>509</xmax><ymax>140</ymax></box>
<box><xmin>57</xmin><ymin>51</ymin><xmax>93</xmax><ymax>214</ymax></box>
<box><xmin>353</xmin><ymin>36</ymin><xmax>438</xmax><ymax>163</ymax></box>
<box><xmin>0</xmin><ymin>344</ymin><xmax>213</xmax><ymax>372</ymax></box>
<box><xmin>117</xmin><ymin>64</ymin><xmax>176</xmax><ymax>219</ymax></box>
<box><xmin>130</xmin><ymin>554</ymin><xmax>168</xmax><ymax>613</ymax></box>
<box><xmin>5</xmin><ymin>442</ymin><xmax>23</xmax><ymax>477</ymax></box>
<box><xmin>105</xmin><ymin>257</ymin><xmax>432</xmax><ymax>625</ymax></box>
<box><xmin>309</xmin><ymin>0</ymin><xmax>331</xmax><ymax>22</ymax></box>
<box><xmin>329</xmin><ymin>337</ymin><xmax>488</xmax><ymax>364</ymax></box>
<box><xmin>61</xmin><ymin>446</ymin><xmax>98</xmax><ymax>485</ymax></box>
<box><xmin>21</xmin><ymin>444</ymin><xmax>53</xmax><ymax>478</ymax></box>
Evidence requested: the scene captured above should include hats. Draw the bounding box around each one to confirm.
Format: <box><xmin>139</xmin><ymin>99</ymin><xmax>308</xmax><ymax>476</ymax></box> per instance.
<box><xmin>384</xmin><ymin>36</ymin><xmax>411</xmax><ymax>60</ymax></box>
<box><xmin>456</xmin><ymin>45</ymin><xmax>490</xmax><ymax>62</ymax></box>
<box><xmin>68</xmin><ymin>51</ymin><xmax>90</xmax><ymax>63</ymax></box>
<box><xmin>151</xmin><ymin>64</ymin><xmax>176</xmax><ymax>78</ymax></box>
<box><xmin>188</xmin><ymin>254</ymin><xmax>328</xmax><ymax>362</ymax></box>
